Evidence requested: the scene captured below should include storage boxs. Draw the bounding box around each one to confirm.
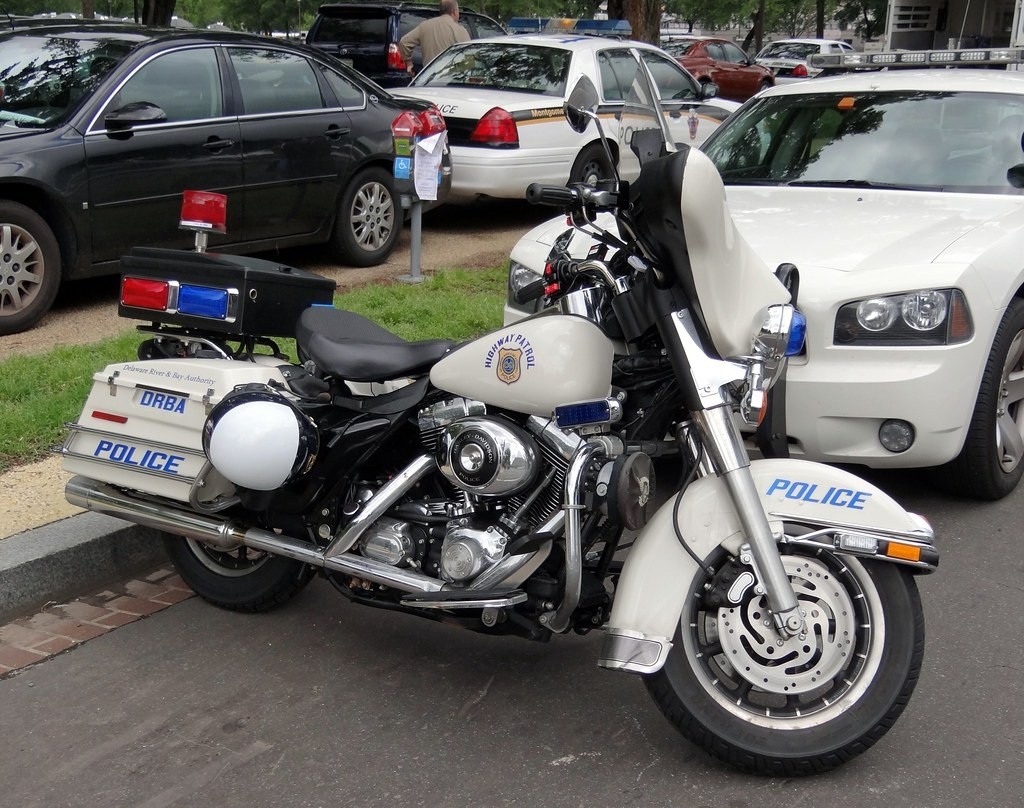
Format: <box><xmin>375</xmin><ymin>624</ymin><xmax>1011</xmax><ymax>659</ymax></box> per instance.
<box><xmin>117</xmin><ymin>246</ymin><xmax>336</xmax><ymax>339</ymax></box>
<box><xmin>61</xmin><ymin>358</ymin><xmax>294</xmax><ymax>505</ymax></box>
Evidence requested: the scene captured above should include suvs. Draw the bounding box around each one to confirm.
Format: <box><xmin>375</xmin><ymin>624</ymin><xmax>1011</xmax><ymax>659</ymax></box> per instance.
<box><xmin>303</xmin><ymin>0</ymin><xmax>509</xmax><ymax>89</ymax></box>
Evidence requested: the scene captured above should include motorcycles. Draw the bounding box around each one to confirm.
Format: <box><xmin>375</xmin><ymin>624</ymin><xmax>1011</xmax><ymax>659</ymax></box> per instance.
<box><xmin>59</xmin><ymin>56</ymin><xmax>940</xmax><ymax>779</ymax></box>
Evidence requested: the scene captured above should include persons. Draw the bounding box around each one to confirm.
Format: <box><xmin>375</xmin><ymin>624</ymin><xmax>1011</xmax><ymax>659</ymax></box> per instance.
<box><xmin>398</xmin><ymin>0</ymin><xmax>472</xmax><ymax>77</ymax></box>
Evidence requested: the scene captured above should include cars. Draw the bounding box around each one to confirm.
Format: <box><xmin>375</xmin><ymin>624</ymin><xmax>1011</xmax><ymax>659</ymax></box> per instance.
<box><xmin>382</xmin><ymin>17</ymin><xmax>770</xmax><ymax>213</ymax></box>
<box><xmin>753</xmin><ymin>38</ymin><xmax>856</xmax><ymax>86</ymax></box>
<box><xmin>658</xmin><ymin>38</ymin><xmax>776</xmax><ymax>103</ymax></box>
<box><xmin>503</xmin><ymin>47</ymin><xmax>1024</xmax><ymax>504</ymax></box>
<box><xmin>0</xmin><ymin>24</ymin><xmax>454</xmax><ymax>336</ymax></box>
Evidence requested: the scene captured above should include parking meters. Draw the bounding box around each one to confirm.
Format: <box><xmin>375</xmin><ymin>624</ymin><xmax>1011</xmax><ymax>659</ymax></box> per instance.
<box><xmin>391</xmin><ymin>109</ymin><xmax>447</xmax><ymax>284</ymax></box>
